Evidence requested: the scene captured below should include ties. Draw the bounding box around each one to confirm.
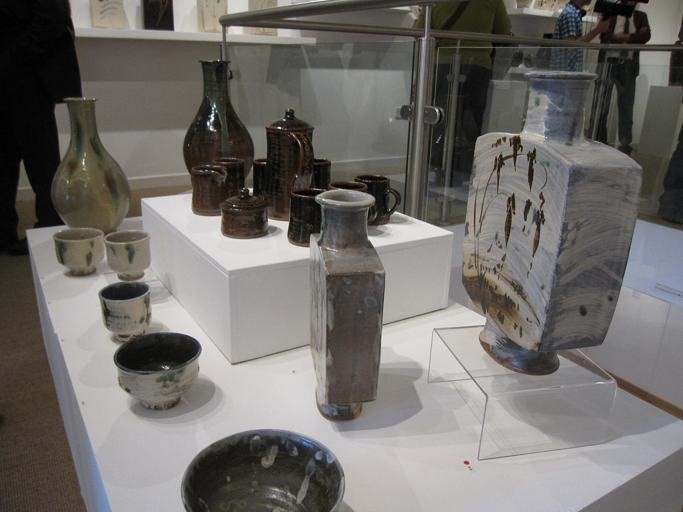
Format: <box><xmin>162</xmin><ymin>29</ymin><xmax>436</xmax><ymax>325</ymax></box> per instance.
<box><xmin>620</xmin><ymin>17</ymin><xmax>630</xmax><ymax>58</ymax></box>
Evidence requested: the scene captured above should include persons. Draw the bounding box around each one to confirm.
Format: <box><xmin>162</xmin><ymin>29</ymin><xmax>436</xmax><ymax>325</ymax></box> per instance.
<box><xmin>657</xmin><ymin>124</ymin><xmax>683</xmax><ymax>225</ymax></box>
<box><xmin>407</xmin><ymin>0</ymin><xmax>514</xmax><ymax>188</ymax></box>
<box><xmin>587</xmin><ymin>1</ymin><xmax>650</xmax><ymax>155</ymax></box>
<box><xmin>0</xmin><ymin>1</ymin><xmax>83</xmax><ymax>257</ymax></box>
<box><xmin>666</xmin><ymin>18</ymin><xmax>683</xmax><ymax>89</ymax></box>
<box><xmin>540</xmin><ymin>0</ymin><xmax>611</xmax><ymax>146</ymax></box>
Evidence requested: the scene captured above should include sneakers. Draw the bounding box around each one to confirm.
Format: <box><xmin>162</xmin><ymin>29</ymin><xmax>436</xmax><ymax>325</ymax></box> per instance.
<box><xmin>7</xmin><ymin>237</ymin><xmax>29</xmax><ymax>257</ymax></box>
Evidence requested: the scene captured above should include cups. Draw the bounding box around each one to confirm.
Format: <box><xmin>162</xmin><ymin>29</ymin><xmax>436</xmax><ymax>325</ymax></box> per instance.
<box><xmin>111</xmin><ymin>329</ymin><xmax>204</xmax><ymax>414</ymax></box>
<box><xmin>262</xmin><ymin>105</ymin><xmax>315</xmax><ymax>221</ymax></box>
<box><xmin>101</xmin><ymin>229</ymin><xmax>155</xmax><ymax>282</ymax></box>
<box><xmin>95</xmin><ymin>283</ymin><xmax>156</xmax><ymax>344</ymax></box>
<box><xmin>51</xmin><ymin>227</ymin><xmax>104</xmax><ymax>276</ymax></box>
<box><xmin>180</xmin><ymin>424</ymin><xmax>345</xmax><ymax>512</ymax></box>
<box><xmin>189</xmin><ymin>153</ymin><xmax>403</xmax><ymax>249</ymax></box>
